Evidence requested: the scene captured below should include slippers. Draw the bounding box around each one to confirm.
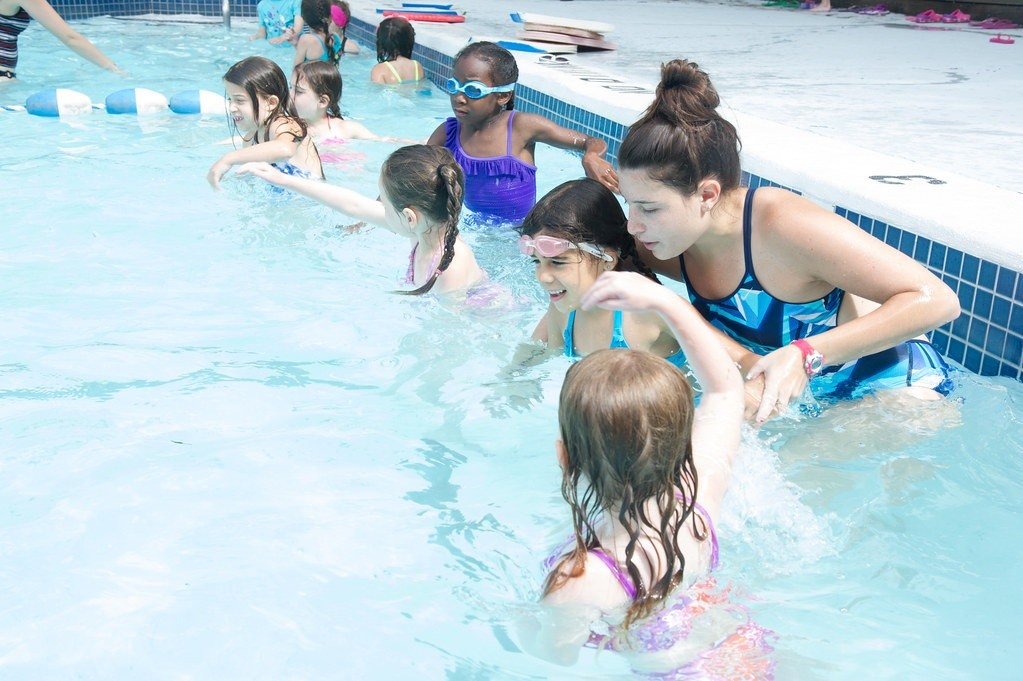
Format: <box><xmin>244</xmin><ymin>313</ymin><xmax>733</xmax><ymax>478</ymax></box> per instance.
<box><xmin>916</xmin><ymin>9</ymin><xmax>944</xmax><ymax>23</ymax></box>
<box><xmin>837</xmin><ymin>0</ymin><xmax>890</xmax><ymax>16</ymax></box>
<box><xmin>941</xmin><ymin>9</ymin><xmax>971</xmax><ymax>23</ymax></box>
<box><xmin>969</xmin><ymin>17</ymin><xmax>1018</xmax><ymax>29</ymax></box>
<box><xmin>989</xmin><ymin>33</ymin><xmax>1014</xmax><ymax>44</ymax></box>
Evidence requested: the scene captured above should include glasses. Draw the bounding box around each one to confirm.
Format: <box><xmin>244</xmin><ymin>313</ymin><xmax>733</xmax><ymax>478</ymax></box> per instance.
<box><xmin>517</xmin><ymin>235</ymin><xmax>614</xmax><ymax>262</ymax></box>
<box><xmin>445</xmin><ymin>77</ymin><xmax>493</xmax><ymax>99</ymax></box>
<box><xmin>537</xmin><ymin>54</ymin><xmax>570</xmax><ymax>64</ymax></box>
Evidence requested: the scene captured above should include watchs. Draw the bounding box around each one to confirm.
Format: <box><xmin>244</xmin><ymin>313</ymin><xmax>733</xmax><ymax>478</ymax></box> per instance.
<box><xmin>790</xmin><ymin>339</ymin><xmax>823</xmax><ymax>380</ymax></box>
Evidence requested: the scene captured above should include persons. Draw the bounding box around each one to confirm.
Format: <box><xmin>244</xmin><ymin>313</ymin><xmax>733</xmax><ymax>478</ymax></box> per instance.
<box><xmin>517</xmin><ymin>178</ymin><xmax>765</xmax><ymax>401</ymax></box>
<box><xmin>370</xmin><ymin>17</ymin><xmax>424</xmax><ymax>83</ymax></box>
<box><xmin>536</xmin><ymin>271</ymin><xmax>743</xmax><ymax>605</ymax></box>
<box><xmin>0</xmin><ymin>0</ymin><xmax>115</xmax><ymax>80</ymax></box>
<box><xmin>206</xmin><ymin>55</ymin><xmax>322</xmax><ymax>190</ymax></box>
<box><xmin>290</xmin><ymin>59</ymin><xmax>372</xmax><ymax>140</ymax></box>
<box><xmin>618</xmin><ymin>57</ymin><xmax>961</xmax><ymax>421</ymax></box>
<box><xmin>330</xmin><ymin>1</ymin><xmax>360</xmax><ymax>52</ymax></box>
<box><xmin>425</xmin><ymin>41</ymin><xmax>619</xmax><ymax>220</ymax></box>
<box><xmin>235</xmin><ymin>143</ymin><xmax>480</xmax><ymax>294</ymax></box>
<box><xmin>286</xmin><ymin>0</ymin><xmax>341</xmax><ymax>68</ymax></box>
<box><xmin>250</xmin><ymin>0</ymin><xmax>305</xmax><ymax>46</ymax></box>
